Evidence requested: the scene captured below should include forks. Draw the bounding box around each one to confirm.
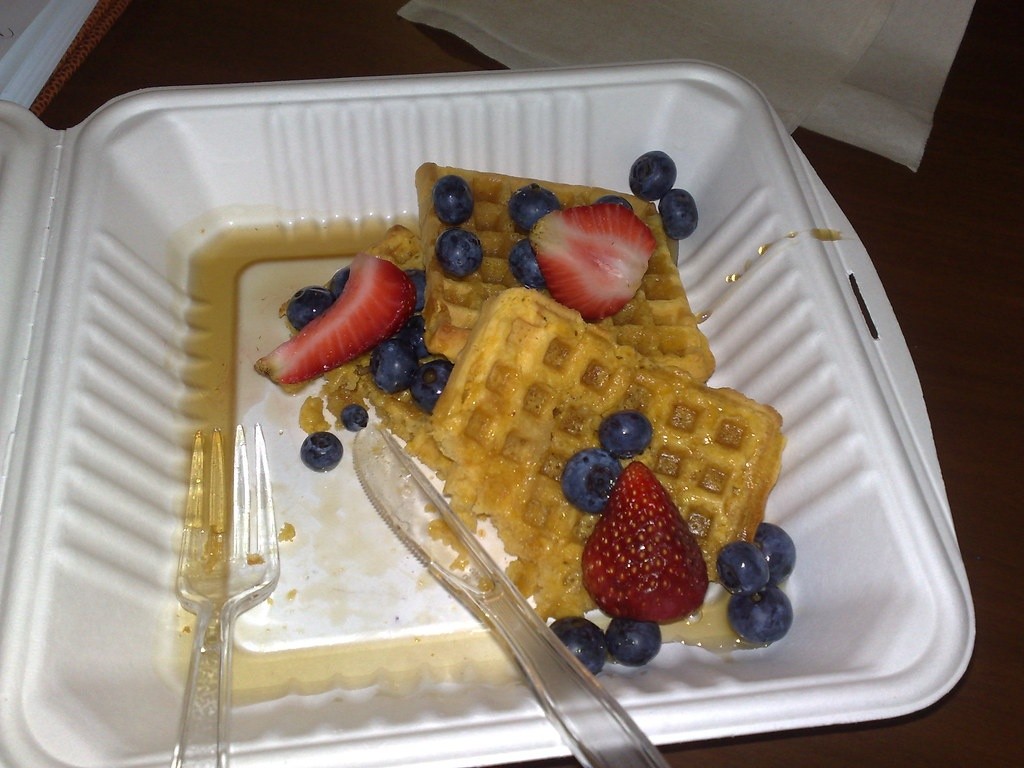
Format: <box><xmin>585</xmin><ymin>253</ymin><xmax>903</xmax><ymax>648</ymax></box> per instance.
<box><xmin>171</xmin><ymin>422</ymin><xmax>281</xmax><ymax>768</ymax></box>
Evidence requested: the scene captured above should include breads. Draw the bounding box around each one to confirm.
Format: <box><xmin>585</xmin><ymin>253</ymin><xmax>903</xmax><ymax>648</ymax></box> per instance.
<box><xmin>312</xmin><ymin>162</ymin><xmax>787</xmax><ymax>625</ymax></box>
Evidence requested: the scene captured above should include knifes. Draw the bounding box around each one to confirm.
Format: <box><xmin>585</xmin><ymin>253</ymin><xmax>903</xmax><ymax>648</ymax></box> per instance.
<box><xmin>352</xmin><ymin>419</ymin><xmax>670</xmax><ymax>768</ymax></box>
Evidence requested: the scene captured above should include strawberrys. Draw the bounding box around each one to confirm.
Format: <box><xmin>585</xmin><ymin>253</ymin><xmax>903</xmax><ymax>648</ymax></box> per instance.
<box><xmin>254</xmin><ymin>253</ymin><xmax>417</xmax><ymax>383</ymax></box>
<box><xmin>581</xmin><ymin>460</ymin><xmax>709</xmax><ymax>623</ymax></box>
<box><xmin>529</xmin><ymin>202</ymin><xmax>658</xmax><ymax>324</ymax></box>
